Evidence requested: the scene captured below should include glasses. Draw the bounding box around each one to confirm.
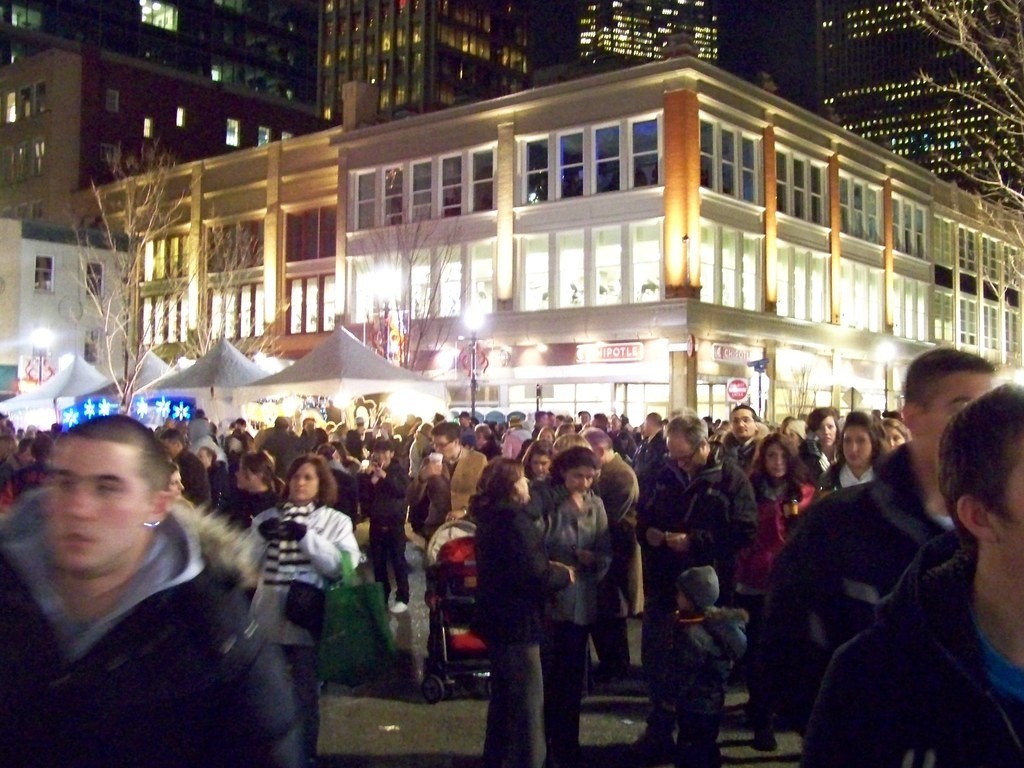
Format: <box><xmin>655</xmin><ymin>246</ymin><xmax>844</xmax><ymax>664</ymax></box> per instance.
<box><xmin>432</xmin><ymin>439</ymin><xmax>459</xmax><ymax>448</ymax></box>
<box><xmin>672</xmin><ymin>445</ymin><xmax>700</xmax><ymax>463</ymax></box>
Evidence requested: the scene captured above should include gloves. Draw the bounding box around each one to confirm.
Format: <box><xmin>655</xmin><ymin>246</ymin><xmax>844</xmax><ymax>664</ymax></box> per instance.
<box><xmin>257</xmin><ymin>517</ymin><xmax>307</xmax><ymax>542</ymax></box>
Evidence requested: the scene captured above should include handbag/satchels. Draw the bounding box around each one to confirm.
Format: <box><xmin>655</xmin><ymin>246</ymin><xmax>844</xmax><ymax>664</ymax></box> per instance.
<box><xmin>315</xmin><ymin>550</ymin><xmax>397</xmax><ymax>686</ymax></box>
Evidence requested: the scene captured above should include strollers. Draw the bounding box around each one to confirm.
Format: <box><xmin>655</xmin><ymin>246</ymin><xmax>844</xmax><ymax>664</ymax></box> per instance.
<box><xmin>421</xmin><ymin>519</ymin><xmax>493</xmax><ymax>705</ymax></box>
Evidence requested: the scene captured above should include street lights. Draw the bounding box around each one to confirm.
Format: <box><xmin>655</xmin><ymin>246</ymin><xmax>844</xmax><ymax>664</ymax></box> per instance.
<box><xmin>876</xmin><ymin>341</ymin><xmax>898</xmax><ymax>410</ymax></box>
<box><xmin>31</xmin><ymin>328</ymin><xmax>52</xmax><ymax>385</ymax></box>
<box><xmin>462</xmin><ymin>306</ymin><xmax>487</xmax><ymax>420</ymax></box>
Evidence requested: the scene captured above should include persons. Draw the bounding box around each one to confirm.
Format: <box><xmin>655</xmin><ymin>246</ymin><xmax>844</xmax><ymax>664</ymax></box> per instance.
<box><xmin>0</xmin><ymin>349</ymin><xmax>1024</xmax><ymax>768</ymax></box>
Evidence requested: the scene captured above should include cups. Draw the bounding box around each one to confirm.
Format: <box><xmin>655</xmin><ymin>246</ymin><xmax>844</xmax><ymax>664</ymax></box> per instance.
<box><xmin>429</xmin><ymin>452</ymin><xmax>444</xmax><ymax>466</ymax></box>
<box><xmin>362</xmin><ymin>459</ymin><xmax>369</xmax><ymax>470</ymax></box>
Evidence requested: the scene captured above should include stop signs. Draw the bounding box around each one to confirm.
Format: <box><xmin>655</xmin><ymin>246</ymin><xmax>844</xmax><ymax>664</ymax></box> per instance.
<box><xmin>729</xmin><ymin>381</ymin><xmax>747</xmax><ymax>401</ymax></box>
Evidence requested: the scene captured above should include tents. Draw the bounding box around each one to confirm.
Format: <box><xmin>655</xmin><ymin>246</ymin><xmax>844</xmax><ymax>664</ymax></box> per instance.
<box><xmin>247</xmin><ymin>324</ymin><xmax>432</xmax><ymax>391</ymax></box>
<box><xmin>146</xmin><ymin>335</ymin><xmax>272</xmax><ymax>399</ymax></box>
<box><xmin>90</xmin><ymin>347</ymin><xmax>175</xmax><ymax>395</ymax></box>
<box><xmin>1</xmin><ymin>354</ymin><xmax>112</xmax><ymax>424</ymax></box>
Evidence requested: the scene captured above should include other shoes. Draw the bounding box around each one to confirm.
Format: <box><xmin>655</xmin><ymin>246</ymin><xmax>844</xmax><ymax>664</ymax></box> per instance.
<box><xmin>391</xmin><ymin>601</ymin><xmax>408</xmax><ymax>613</ymax></box>
<box><xmin>754</xmin><ymin>725</ymin><xmax>777</xmax><ymax>751</ymax></box>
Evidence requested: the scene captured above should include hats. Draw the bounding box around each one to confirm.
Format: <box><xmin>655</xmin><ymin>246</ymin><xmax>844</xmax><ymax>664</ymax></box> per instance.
<box><xmin>680</xmin><ymin>565</ymin><xmax>720</xmax><ymax>609</ymax></box>
<box><xmin>510</xmin><ymin>416</ymin><xmax>521</xmax><ymax>426</ymax></box>
<box><xmin>355</xmin><ymin>417</ymin><xmax>364</xmax><ymax>424</ymax></box>
